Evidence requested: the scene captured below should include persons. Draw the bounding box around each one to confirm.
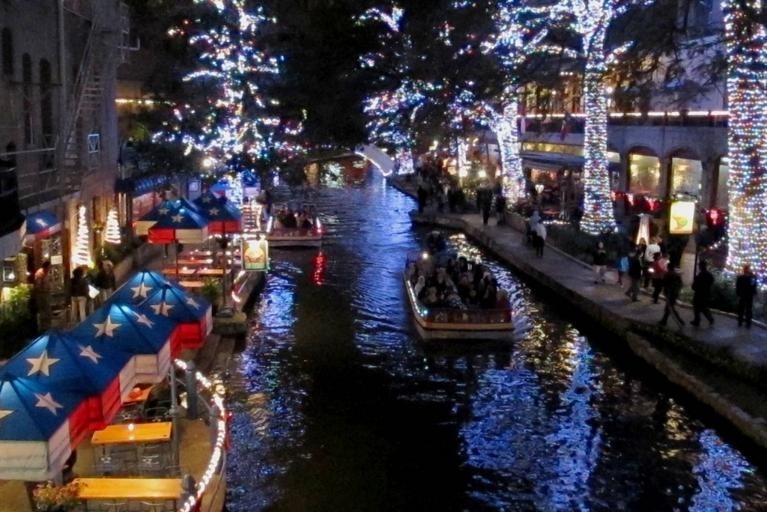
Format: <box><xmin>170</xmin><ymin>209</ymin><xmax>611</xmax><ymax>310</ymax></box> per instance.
<box><xmin>416</xmin><ymin>181</ymin><xmax>506</xmax><ymax>224</ymax></box>
<box><xmin>533</xmin><ymin>218</ymin><xmax>548</xmax><ymax>257</ymax></box>
<box><xmin>71</xmin><ymin>262</ymin><xmax>117</xmax><ymax>321</ymax></box>
<box><xmin>593</xmin><ymin>233</ymin><xmax>667</xmax><ymax>302</ymax></box>
<box><xmin>412</xmin><ymin>228</ymin><xmax>497</xmax><ymax>309</ymax></box>
<box><xmin>735</xmin><ymin>266</ymin><xmax>759</xmax><ymax>330</ymax></box>
<box><xmin>659</xmin><ymin>264</ymin><xmax>686</xmax><ymax>331</ymax></box>
<box><xmin>277</xmin><ymin>205</ymin><xmax>313</xmax><ymax>232</ymax></box>
<box><xmin>689</xmin><ymin>260</ymin><xmax>718</xmax><ymax>327</ymax></box>
<box><xmin>33</xmin><ymin>260</ymin><xmax>50</xmax><ymax>290</ymax></box>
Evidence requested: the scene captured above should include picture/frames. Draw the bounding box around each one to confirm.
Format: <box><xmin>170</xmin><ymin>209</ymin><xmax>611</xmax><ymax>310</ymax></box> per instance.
<box><xmin>3</xmin><ymin>266</ymin><xmax>14</xmax><ymax>283</ymax></box>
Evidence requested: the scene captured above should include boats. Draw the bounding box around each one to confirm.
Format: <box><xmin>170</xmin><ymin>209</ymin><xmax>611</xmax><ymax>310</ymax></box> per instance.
<box><xmin>268</xmin><ymin>200</ymin><xmax>323</xmax><ymax>248</ymax></box>
<box><xmin>401</xmin><ymin>258</ymin><xmax>515</xmax><ymax>341</ymax></box>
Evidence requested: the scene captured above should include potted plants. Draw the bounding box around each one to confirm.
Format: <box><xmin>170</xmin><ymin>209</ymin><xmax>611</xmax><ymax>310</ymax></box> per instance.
<box><xmin>198</xmin><ymin>276</ymin><xmax>224</xmax><ymax>316</ymax></box>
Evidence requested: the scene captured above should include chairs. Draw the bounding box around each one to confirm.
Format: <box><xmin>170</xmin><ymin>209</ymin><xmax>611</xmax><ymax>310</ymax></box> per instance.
<box><xmin>91</xmin><ymin>399</ymin><xmax>190</xmax><ymax>512</ymax></box>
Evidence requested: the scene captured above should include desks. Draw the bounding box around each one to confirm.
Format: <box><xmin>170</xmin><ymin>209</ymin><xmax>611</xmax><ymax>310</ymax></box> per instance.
<box><xmin>159</xmin><ymin>249</ymin><xmax>241</xmax><ymax>293</ymax></box>
<box><xmin>124</xmin><ymin>386</ymin><xmax>154</xmax><ymax>403</ymax></box>
<box><xmin>72</xmin><ymin>477</ymin><xmax>181</xmax><ymax>512</ymax></box>
